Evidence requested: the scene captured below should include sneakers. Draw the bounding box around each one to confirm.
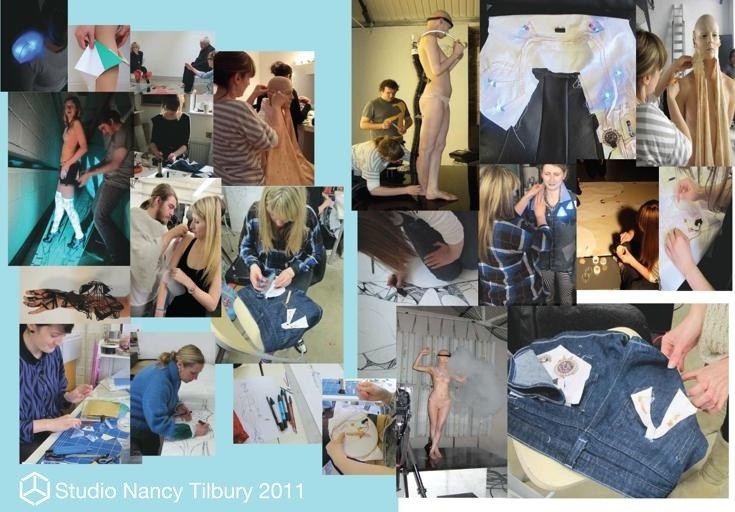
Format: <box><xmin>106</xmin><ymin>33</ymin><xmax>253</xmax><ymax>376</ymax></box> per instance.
<box><xmin>297</xmin><ymin>338</ymin><xmax>307</xmax><ymax>354</ymax></box>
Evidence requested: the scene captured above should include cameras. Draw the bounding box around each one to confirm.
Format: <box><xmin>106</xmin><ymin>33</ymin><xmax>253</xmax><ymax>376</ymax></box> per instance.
<box><xmin>394</xmin><ymin>387</ymin><xmax>411</xmax><ymax>437</ymax></box>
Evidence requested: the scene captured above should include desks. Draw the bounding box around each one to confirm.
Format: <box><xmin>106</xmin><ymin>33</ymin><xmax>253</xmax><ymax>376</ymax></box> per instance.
<box><xmin>226</xmin><ymin>255</ymin><xmax>314</xmax><ymax>296</ymax></box>
<box><xmin>24</xmin><ymin>385</ymin><xmax>130</xmax><ymax>465</ymax></box>
<box><xmin>399</xmin><ymin>445</ymin><xmax>508</xmax><ymax>498</ymax></box>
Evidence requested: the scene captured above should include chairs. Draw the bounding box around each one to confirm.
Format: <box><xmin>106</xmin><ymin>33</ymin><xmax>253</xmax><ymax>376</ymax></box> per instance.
<box><xmin>226</xmin><ymin>202</ymin><xmax>327</xmax><ymax>288</ymax></box>
<box><xmin>212</xmin><ymin>296</ymin><xmax>300</xmax><ymax>363</ymax></box>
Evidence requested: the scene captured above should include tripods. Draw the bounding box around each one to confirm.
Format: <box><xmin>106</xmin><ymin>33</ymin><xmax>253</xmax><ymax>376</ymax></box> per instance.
<box><xmin>395</xmin><ymin>435</ymin><xmax>427</xmax><ymax>499</ymax></box>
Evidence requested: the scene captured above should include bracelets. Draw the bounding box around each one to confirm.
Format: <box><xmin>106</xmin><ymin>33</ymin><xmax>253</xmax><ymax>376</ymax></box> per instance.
<box><xmin>156</xmin><ymin>307</ymin><xmax>166</xmax><ymax>312</ymax></box>
<box><xmin>186</xmin><ymin>284</ymin><xmax>198</xmax><ymax>296</ymax></box>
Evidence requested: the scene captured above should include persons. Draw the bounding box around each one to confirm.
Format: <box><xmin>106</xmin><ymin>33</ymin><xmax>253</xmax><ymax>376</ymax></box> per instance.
<box><xmin>259</xmin><ymin>60</ymin><xmax>310</xmax><ymax>146</ymax></box>
<box><xmin>154</xmin><ymin>195</ymin><xmax>222</xmax><ymax>318</ymax></box>
<box><xmin>415</xmin><ymin>8</ymin><xmax>464</xmax><ymax>203</ymax></box>
<box><xmin>258</xmin><ymin>75</ymin><xmax>315</xmax><ymax>187</ymax></box>
<box><xmin>181</xmin><ymin>35</ymin><xmax>218</xmax><ymax>95</ymax></box>
<box><xmin>77</xmin><ymin>109</ymin><xmax>133</xmax><ymax>266</ymax></box>
<box><xmin>480</xmin><ymin>166</ymin><xmax>548</xmax><ymax>306</ymax></box>
<box><xmin>412</xmin><ymin>347</ymin><xmax>469</xmax><ymax>461</ymax></box>
<box><xmin>671</xmin><ymin>14</ymin><xmax>735</xmax><ymax>166</ymax></box>
<box><xmin>358</xmin><ymin>80</ymin><xmax>411</xmax><ymax>180</ymax></box>
<box><xmin>665</xmin><ymin>177</ymin><xmax>733</xmax><ymax>291</ymax></box>
<box><xmin>19</xmin><ymin>323</ymin><xmax>94</xmax><ymax>464</ymax></box>
<box><xmin>150</xmin><ymin>94</ymin><xmax>192</xmax><ymax>168</ymax></box>
<box><xmin>21</xmin><ymin>287</ymin><xmax>131</xmax><ymax>319</ymax></box>
<box><xmin>358</xmin><ymin>210</ymin><xmax>478</xmax><ymax>289</ymax></box>
<box><xmin>616</xmin><ymin>197</ymin><xmax>659</xmax><ymax>290</ymax></box>
<box><xmin>636</xmin><ymin>29</ymin><xmax>693</xmax><ymax>170</ymax></box>
<box><xmin>238</xmin><ymin>186</ymin><xmax>324</xmax><ymax>355</ymax></box>
<box><xmin>130</xmin><ymin>343</ymin><xmax>210</xmax><ymax>455</ymax></box>
<box><xmin>325</xmin><ymin>381</ymin><xmax>401</xmax><ymax>476</ymax></box>
<box><xmin>353</xmin><ymin>130</ymin><xmax>425</xmax><ymax>198</ymax></box>
<box><xmin>213</xmin><ymin>51</ymin><xmax>278</xmax><ymax>186</ymax></box>
<box><xmin>129</xmin><ymin>42</ymin><xmax>152</xmax><ymax>93</ymax></box>
<box><xmin>525</xmin><ymin>165</ymin><xmax>577</xmax><ymax>307</ymax></box>
<box><xmin>659</xmin><ymin>303</ymin><xmax>729</xmax><ymax>418</ymax></box>
<box><xmin>42</xmin><ymin>97</ymin><xmax>89</xmax><ymax>249</ymax></box>
<box><xmin>75</xmin><ymin>24</ymin><xmax>129</xmax><ymax>93</ymax></box>
<box><xmin>130</xmin><ymin>182</ymin><xmax>189</xmax><ymax>316</ymax></box>
<box><xmin>721</xmin><ymin>48</ymin><xmax>735</xmax><ymax>79</ymax></box>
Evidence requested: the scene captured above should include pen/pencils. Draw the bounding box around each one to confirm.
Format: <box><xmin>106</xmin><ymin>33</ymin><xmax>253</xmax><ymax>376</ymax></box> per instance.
<box><xmin>266</xmin><ymin>388</ymin><xmax>296</xmax><ymax>432</ymax></box>
<box><xmin>174</xmin><ymin>411</ymin><xmax>192</xmax><ymax>416</ymax></box>
<box><xmin>199</xmin><ymin>421</ymin><xmax>205</xmax><ymax>425</ymax></box>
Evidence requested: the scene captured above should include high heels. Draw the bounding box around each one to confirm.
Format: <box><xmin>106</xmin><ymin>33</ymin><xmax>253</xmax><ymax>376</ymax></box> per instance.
<box><xmin>67</xmin><ymin>235</ymin><xmax>86</xmax><ymax>249</ymax></box>
<box><xmin>43</xmin><ymin>230</ymin><xmax>60</xmax><ymax>243</ymax></box>
